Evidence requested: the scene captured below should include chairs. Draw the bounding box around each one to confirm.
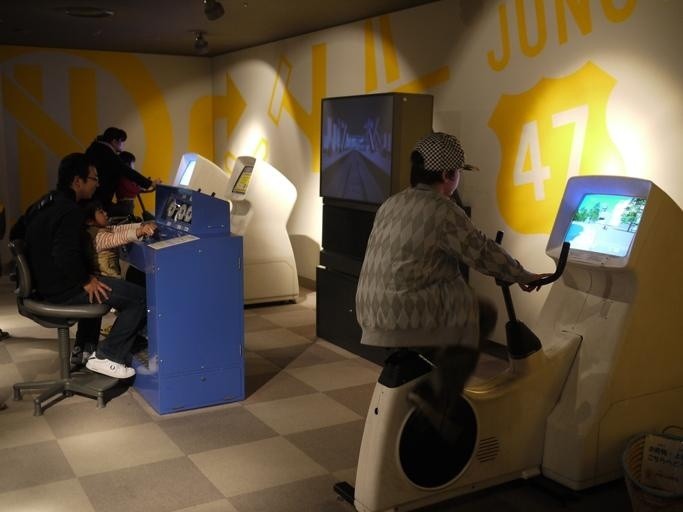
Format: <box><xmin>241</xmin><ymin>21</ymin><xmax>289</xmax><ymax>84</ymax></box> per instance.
<box><xmin>7</xmin><ymin>240</ymin><xmax>118</xmax><ymax>417</ymax></box>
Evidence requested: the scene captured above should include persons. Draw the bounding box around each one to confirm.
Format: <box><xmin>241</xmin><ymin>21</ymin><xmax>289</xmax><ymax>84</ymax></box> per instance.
<box><xmin>352</xmin><ymin>132</ymin><xmax>554</xmax><ymax>443</ymax></box>
<box><xmin>11</xmin><ymin>124</ymin><xmax>164</xmax><ymax>381</ymax></box>
<box><xmin>82</xmin><ymin>126</ymin><xmax>161</xmax><ymax>227</ymax></box>
<box><xmin>69</xmin><ymin>198</ymin><xmax>159</xmax><ymax>367</ymax></box>
<box><xmin>114</xmin><ymin>151</ymin><xmax>151</xmax><ymax>217</ymax></box>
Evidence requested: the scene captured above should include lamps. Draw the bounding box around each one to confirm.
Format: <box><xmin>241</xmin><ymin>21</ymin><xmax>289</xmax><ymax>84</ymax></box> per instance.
<box><xmin>192</xmin><ymin>30</ymin><xmax>208</xmax><ymax>49</ymax></box>
<box><xmin>204</xmin><ymin>0</ymin><xmax>224</xmax><ymax>21</ymax></box>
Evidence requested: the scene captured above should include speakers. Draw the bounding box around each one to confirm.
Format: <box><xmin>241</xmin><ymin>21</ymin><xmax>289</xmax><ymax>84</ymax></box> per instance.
<box><xmin>312</xmin><ymin>265</ymin><xmax>412</xmax><ymax>372</ymax></box>
<box><xmin>320</xmin><ymin>204</ymin><xmax>375</xmax><ymax>268</ymax></box>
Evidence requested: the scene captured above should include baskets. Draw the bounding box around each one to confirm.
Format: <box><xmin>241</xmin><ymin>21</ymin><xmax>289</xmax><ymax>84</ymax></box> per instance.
<box><xmin>622</xmin><ymin>426</ymin><xmax>683</xmax><ymax>512</ymax></box>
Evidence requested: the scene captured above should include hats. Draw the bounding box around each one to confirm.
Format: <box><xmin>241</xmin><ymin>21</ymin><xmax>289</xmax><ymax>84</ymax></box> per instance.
<box><xmin>416</xmin><ymin>133</ymin><xmax>472</xmax><ymax>172</ymax></box>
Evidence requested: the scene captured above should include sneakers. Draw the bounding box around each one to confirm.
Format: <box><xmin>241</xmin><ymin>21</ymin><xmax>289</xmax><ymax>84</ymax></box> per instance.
<box><xmin>71</xmin><ymin>346</ymin><xmax>136</xmax><ymax>379</ymax></box>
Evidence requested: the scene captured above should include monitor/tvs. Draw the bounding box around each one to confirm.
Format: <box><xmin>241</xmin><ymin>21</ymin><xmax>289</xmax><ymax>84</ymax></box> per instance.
<box><xmin>178</xmin><ymin>158</ymin><xmax>195</xmax><ymax>189</ymax></box>
<box><xmin>558</xmin><ymin>188</ymin><xmax>649</xmax><ymax>260</ymax></box>
<box><xmin>230</xmin><ymin>162</ymin><xmax>255</xmax><ymax>195</ymax></box>
